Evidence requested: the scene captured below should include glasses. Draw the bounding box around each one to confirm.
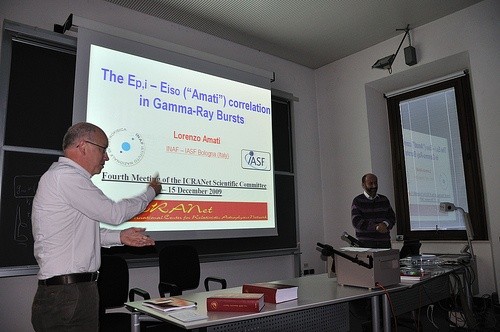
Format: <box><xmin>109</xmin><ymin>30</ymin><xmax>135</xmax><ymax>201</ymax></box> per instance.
<box><xmin>76</xmin><ymin>141</ymin><xmax>108</xmax><ymax>154</ymax></box>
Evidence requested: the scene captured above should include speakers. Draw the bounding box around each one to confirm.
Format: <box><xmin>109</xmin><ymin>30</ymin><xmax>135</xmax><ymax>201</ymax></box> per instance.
<box><xmin>404</xmin><ymin>46</ymin><xmax>417</xmax><ymax>66</ymax></box>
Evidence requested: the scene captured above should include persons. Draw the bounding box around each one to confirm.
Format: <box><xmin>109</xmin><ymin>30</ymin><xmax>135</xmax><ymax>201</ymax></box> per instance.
<box><xmin>31</xmin><ymin>122</ymin><xmax>162</xmax><ymax>332</ymax></box>
<box><xmin>351</xmin><ymin>174</ymin><xmax>396</xmax><ymax>248</ymax></box>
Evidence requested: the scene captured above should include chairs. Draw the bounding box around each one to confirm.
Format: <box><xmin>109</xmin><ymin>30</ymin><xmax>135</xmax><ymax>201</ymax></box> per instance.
<box><xmin>96</xmin><ymin>256</ymin><xmax>152</xmax><ymax>332</ymax></box>
<box><xmin>158</xmin><ymin>245</ymin><xmax>226</xmax><ymax>298</ymax></box>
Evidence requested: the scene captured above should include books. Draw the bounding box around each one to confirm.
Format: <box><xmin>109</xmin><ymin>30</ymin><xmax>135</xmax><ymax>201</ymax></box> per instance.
<box><xmin>242</xmin><ymin>282</ymin><xmax>299</xmax><ymax>304</ymax></box>
<box><xmin>206</xmin><ymin>294</ymin><xmax>266</xmax><ymax>312</ymax></box>
<box><xmin>142</xmin><ymin>296</ymin><xmax>198</xmax><ymax>312</ymax></box>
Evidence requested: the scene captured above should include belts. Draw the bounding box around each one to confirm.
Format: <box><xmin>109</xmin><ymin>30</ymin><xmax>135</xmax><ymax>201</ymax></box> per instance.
<box><xmin>39</xmin><ymin>271</ymin><xmax>99</xmax><ymax>286</ymax></box>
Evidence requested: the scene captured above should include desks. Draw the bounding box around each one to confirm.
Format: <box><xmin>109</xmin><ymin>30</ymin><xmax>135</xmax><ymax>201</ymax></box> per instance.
<box><xmin>123</xmin><ymin>254</ymin><xmax>479</xmax><ymax>332</ymax></box>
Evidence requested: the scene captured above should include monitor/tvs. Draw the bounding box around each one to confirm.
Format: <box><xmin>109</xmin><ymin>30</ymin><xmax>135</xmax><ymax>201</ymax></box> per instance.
<box><xmin>399</xmin><ymin>239</ymin><xmax>422</xmax><ymax>259</ymax></box>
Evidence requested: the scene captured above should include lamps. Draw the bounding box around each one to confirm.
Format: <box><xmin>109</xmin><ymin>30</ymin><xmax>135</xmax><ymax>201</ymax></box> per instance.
<box><xmin>371</xmin><ymin>25</ymin><xmax>411</xmax><ymax>74</ymax></box>
